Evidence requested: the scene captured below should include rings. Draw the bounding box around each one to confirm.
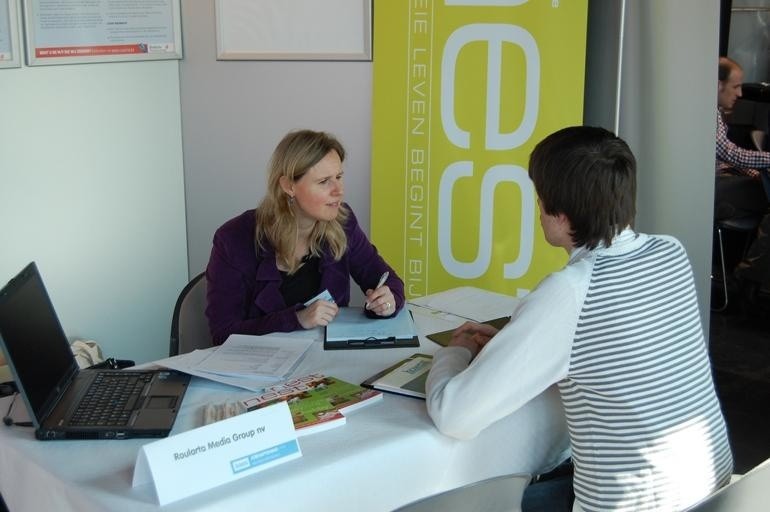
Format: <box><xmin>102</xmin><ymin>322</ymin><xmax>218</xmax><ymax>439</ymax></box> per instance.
<box><xmin>384</xmin><ymin>301</ymin><xmax>391</xmax><ymax>311</ymax></box>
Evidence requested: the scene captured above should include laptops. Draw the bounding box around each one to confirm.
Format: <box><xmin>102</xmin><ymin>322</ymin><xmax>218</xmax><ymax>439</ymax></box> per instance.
<box><xmin>0</xmin><ymin>262</ymin><xmax>190</xmax><ymax>441</ymax></box>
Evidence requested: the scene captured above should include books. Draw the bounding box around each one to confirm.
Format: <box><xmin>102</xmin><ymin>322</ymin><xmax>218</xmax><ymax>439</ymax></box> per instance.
<box><xmin>239</xmin><ymin>379</ymin><xmax>347</xmax><ymax>438</ymax></box>
<box><xmin>264</xmin><ymin>372</ymin><xmax>383</xmax><ymax>417</ymax></box>
<box><xmin>360</xmin><ymin>353</ymin><xmax>434</xmax><ymax>400</ymax></box>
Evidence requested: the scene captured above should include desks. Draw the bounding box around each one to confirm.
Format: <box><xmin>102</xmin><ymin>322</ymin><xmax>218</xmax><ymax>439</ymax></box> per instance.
<box><xmin>1</xmin><ymin>285</ymin><xmax>571</xmax><ymax>511</ymax></box>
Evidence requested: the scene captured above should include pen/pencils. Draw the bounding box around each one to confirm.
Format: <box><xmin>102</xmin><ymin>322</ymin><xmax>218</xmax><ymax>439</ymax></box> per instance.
<box><xmin>366</xmin><ymin>272</ymin><xmax>389</xmax><ymax>309</ymax></box>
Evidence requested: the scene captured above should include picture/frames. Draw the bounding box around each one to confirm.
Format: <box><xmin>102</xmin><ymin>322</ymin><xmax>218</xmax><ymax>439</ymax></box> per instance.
<box><xmin>1</xmin><ymin>2</ymin><xmax>20</xmax><ymax>68</ymax></box>
<box><xmin>23</xmin><ymin>1</ymin><xmax>187</xmax><ymax>67</ymax></box>
<box><xmin>215</xmin><ymin>1</ymin><xmax>374</xmax><ymax>64</ymax></box>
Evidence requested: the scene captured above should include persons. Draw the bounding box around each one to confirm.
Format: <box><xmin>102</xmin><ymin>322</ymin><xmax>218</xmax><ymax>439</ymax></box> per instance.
<box><xmin>205</xmin><ymin>129</ymin><xmax>406</xmax><ymax>345</ymax></box>
<box><xmin>425</xmin><ymin>125</ymin><xmax>735</xmax><ymax>512</ymax></box>
<box><xmin>714</xmin><ymin>57</ymin><xmax>770</xmax><ymax>315</ymax></box>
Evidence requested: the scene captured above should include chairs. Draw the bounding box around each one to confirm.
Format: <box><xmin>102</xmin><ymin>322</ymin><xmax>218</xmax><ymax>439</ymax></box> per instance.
<box><xmin>170</xmin><ymin>271</ymin><xmax>214</xmax><ymax>355</ymax></box>
<box><xmin>710</xmin><ymin>215</ymin><xmax>758</xmax><ymax>313</ymax></box>
<box><xmin>680</xmin><ymin>458</ymin><xmax>770</xmax><ymax>512</ymax></box>
<box><xmin>392</xmin><ymin>474</ymin><xmax>533</xmax><ymax>510</ymax></box>
<box><xmin>745</xmin><ymin>130</ymin><xmax>770</xmax><ymax>293</ymax></box>
